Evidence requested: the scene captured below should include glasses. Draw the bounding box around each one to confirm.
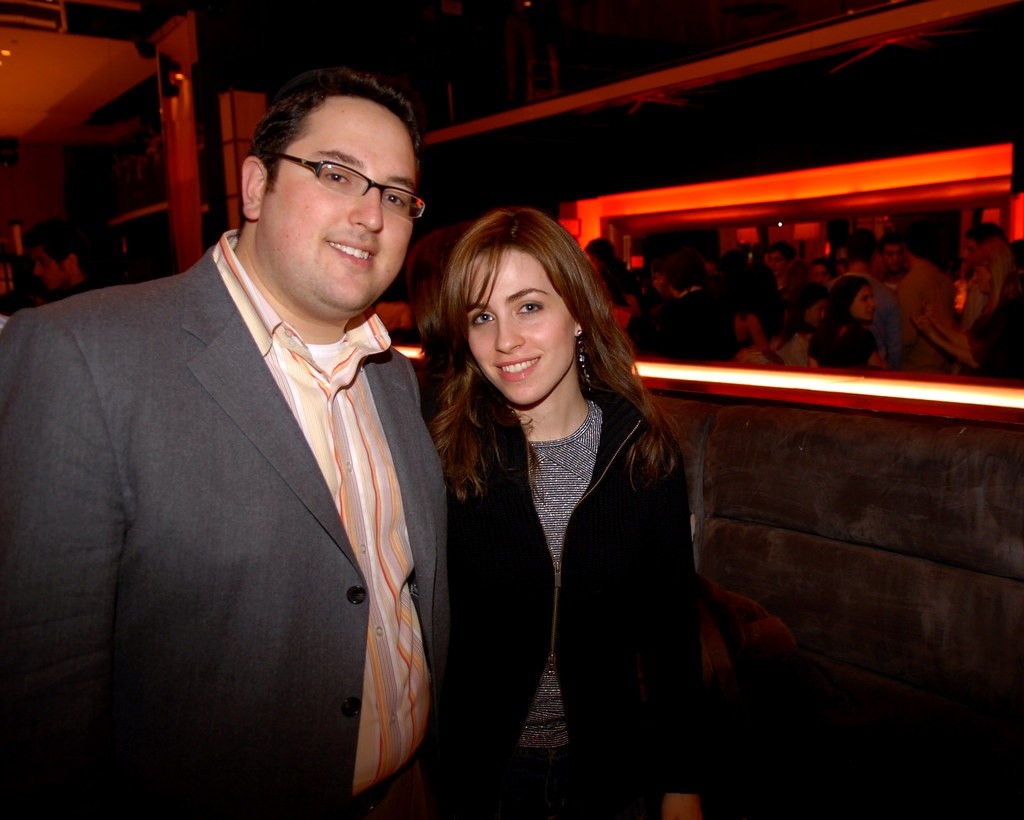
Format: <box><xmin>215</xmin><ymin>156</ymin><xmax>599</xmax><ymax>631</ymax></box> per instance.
<box><xmin>260</xmin><ymin>149</ymin><xmax>426</xmax><ymax>219</ymax></box>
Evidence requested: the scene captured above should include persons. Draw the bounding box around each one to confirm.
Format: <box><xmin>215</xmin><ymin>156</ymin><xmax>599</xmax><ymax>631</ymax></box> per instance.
<box><xmin>0</xmin><ymin>67</ymin><xmax>449</xmax><ymax>820</ymax></box>
<box><xmin>583</xmin><ymin>223</ymin><xmax>1024</xmax><ymax>380</ymax></box>
<box><xmin>423</xmin><ymin>209</ymin><xmax>703</xmax><ymax>820</ymax></box>
<box><xmin>22</xmin><ymin>217</ymin><xmax>102</xmax><ymax>301</ymax></box>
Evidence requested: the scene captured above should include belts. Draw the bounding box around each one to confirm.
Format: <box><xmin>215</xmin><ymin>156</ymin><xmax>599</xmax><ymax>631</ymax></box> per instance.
<box><xmin>347</xmin><ymin>781</ymin><xmax>384</xmax><ymax>814</ymax></box>
